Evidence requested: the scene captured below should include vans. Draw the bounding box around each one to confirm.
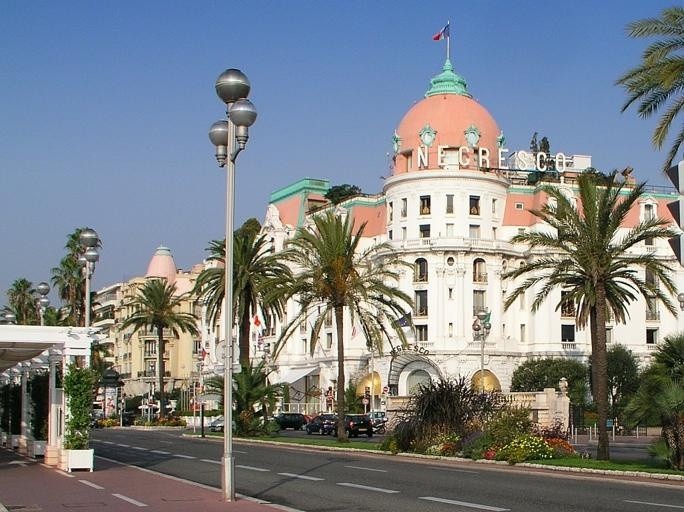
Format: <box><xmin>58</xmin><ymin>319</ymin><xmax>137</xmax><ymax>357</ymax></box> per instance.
<box><xmin>210</xmin><ymin>415</ymin><xmax>237</xmax><ymax>433</ymax></box>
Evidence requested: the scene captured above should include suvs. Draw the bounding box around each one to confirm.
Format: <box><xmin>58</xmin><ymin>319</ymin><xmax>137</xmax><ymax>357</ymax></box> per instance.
<box><xmin>306</xmin><ymin>413</ymin><xmax>339</xmax><ymax>436</ymax></box>
<box><xmin>332</xmin><ymin>413</ymin><xmax>374</xmax><ymax>439</ymax></box>
<box><xmin>270</xmin><ymin>413</ymin><xmax>307</xmax><ymax>431</ymax></box>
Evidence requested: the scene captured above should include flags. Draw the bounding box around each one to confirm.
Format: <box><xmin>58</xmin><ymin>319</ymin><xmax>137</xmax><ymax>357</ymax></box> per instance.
<box><xmin>200</xmin><ymin>348</ymin><xmax>207</xmax><ymax>361</ymax></box>
<box><xmin>431</xmin><ymin>18</ymin><xmax>452</xmax><ymax>46</ymax></box>
<box><xmin>388</xmin><ymin>311</ymin><xmax>413</xmax><ymax>331</ymax></box>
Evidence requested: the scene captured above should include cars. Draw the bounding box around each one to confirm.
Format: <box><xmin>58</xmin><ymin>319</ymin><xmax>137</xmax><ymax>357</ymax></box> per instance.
<box><xmin>367</xmin><ymin>409</ymin><xmax>385</xmax><ymax>419</ymax></box>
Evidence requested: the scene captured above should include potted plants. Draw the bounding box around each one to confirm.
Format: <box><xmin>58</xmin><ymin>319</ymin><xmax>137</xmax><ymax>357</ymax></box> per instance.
<box><xmin>0</xmin><ymin>370</ymin><xmax>49</xmax><ymax>458</ymax></box>
<box><xmin>61</xmin><ymin>361</ymin><xmax>104</xmax><ymax>474</ymax></box>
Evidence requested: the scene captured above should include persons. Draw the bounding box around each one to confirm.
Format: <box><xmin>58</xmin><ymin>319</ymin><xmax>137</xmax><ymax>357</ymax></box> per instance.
<box><xmin>324</xmin><ymin>386</ymin><xmax>334</xmax><ymax>411</ymax></box>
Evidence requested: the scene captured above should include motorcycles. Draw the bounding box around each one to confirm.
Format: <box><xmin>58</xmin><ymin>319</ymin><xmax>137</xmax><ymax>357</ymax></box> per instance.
<box><xmin>371</xmin><ymin>416</ymin><xmax>388</xmax><ymax>435</ymax></box>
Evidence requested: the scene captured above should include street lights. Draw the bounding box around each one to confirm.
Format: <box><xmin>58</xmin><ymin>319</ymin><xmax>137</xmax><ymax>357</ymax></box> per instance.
<box><xmin>196</xmin><ymin>356</ymin><xmax>205</xmax><ymax>429</ymax></box>
<box><xmin>260</xmin><ymin>345</ymin><xmax>274</xmax><ymax>387</ymax></box>
<box><xmin>4</xmin><ymin>311</ymin><xmax>17</xmax><ymax>324</ymax></box>
<box><xmin>472</xmin><ymin>309</ymin><xmax>492</xmax><ymax>394</ymax></box>
<box><xmin>365</xmin><ymin>323</ymin><xmax>380</xmax><ymax>412</ymax></box>
<box><xmin>79</xmin><ymin>227</ymin><xmax>98</xmax><ymax>327</ymax></box>
<box><xmin>34</xmin><ymin>280</ymin><xmax>51</xmax><ymax>325</ymax></box>
<box><xmin>148</xmin><ymin>362</ymin><xmax>154</xmax><ymax>384</ymax></box>
<box><xmin>207</xmin><ymin>68</ymin><xmax>257</xmax><ymax>507</ymax></box>
